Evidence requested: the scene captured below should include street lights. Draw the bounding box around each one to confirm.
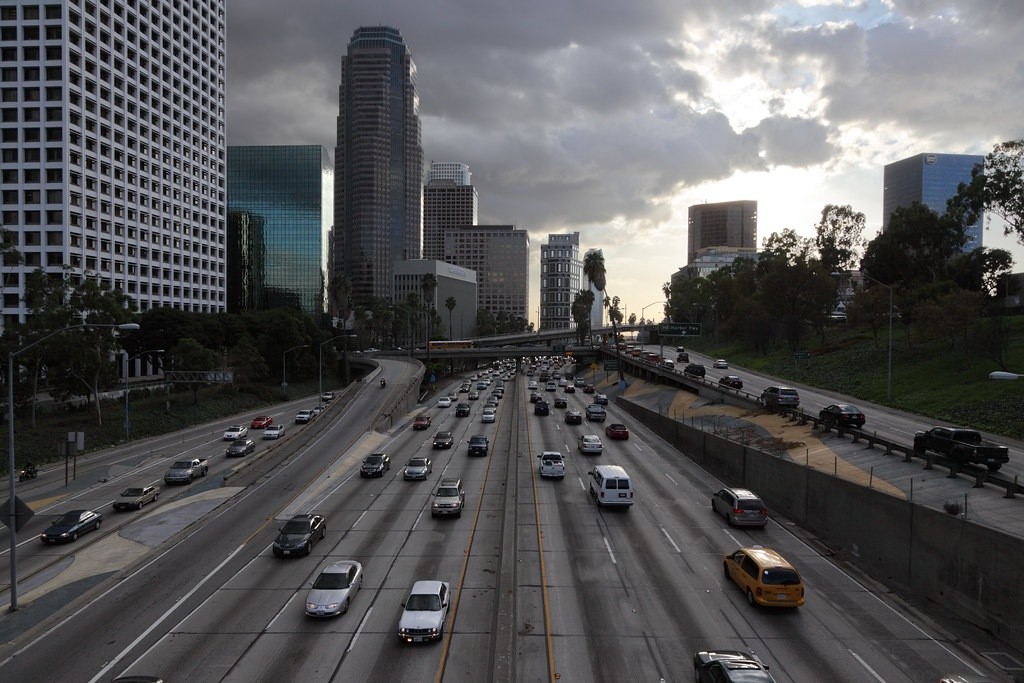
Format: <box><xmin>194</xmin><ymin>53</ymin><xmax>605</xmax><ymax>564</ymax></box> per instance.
<box><xmin>125</xmin><ymin>349</ymin><xmax>166</xmax><ymax>440</ymax></box>
<box><xmin>319</xmin><ymin>333</ymin><xmax>358</xmax><ymax>410</ymax></box>
<box><xmin>693</xmin><ymin>302</ymin><xmax>719</xmax><ymax>355</ymax></box>
<box><xmin>852</xmin><ymin>271</ymin><xmax>893</xmax><ymax>403</ymax></box>
<box><xmin>282</xmin><ymin>344</ymin><xmax>309</xmax><ymax>402</ymax></box>
<box><xmin>9</xmin><ymin>323</ymin><xmax>142</xmax><ymax>608</ymax></box>
<box><xmin>388</xmin><ymin>305</ymin><xmax>411</xmax><ymax>361</ymax></box>
<box><xmin>642</xmin><ymin>300</ymin><xmax>661</xmax><ymax>358</ymax></box>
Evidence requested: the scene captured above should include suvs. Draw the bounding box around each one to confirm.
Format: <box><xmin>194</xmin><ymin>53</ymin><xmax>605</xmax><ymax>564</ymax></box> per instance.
<box><xmin>611</xmin><ymin>343</ymin><xmax>674</xmax><ymax>368</ymax></box>
<box><xmin>431</xmin><ymin>478</ymin><xmax>465</xmax><ymax>517</ymax></box>
<box><xmin>724</xmin><ymin>544</ymin><xmax>806</xmax><ymax>607</ymax></box>
<box><xmin>712</xmin><ymin>487</ymin><xmax>769</xmax><ymax>526</ymax></box>
<box><xmin>467</xmin><ymin>435</ymin><xmax>490</xmax><ymax>457</ymax></box>
<box><xmin>295</xmin><ymin>409</ymin><xmax>314</xmax><ymax>423</ymax></box>
<box><xmin>684</xmin><ymin>363</ymin><xmax>705</xmax><ymax>377</ymax></box>
<box><xmin>761</xmin><ymin>386</ymin><xmax>800</xmax><ymax>407</ymax></box>
<box><xmin>676</xmin><ymin>352</ymin><xmax>689</xmax><ymax>363</ymax></box>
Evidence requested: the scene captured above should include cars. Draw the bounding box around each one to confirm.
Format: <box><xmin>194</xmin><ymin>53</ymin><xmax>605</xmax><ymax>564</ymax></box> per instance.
<box><xmin>274</xmin><ymin>514</ymin><xmax>326</xmax><ymax>557</ymax></box>
<box><xmin>112</xmin><ymin>485</ymin><xmax>161</xmax><ymax>510</ymax></box>
<box><xmin>360</xmin><ymin>453</ymin><xmax>391</xmax><ymax>477</ymax></box>
<box><xmin>606</xmin><ymin>423</ymin><xmax>629</xmax><ymax>440</ymax></box>
<box><xmin>676</xmin><ymin>346</ymin><xmax>684</xmax><ymax>352</ymax></box>
<box><xmin>403</xmin><ymin>457</ymin><xmax>433</xmax><ymax>480</ymax></box>
<box><xmin>251</xmin><ymin>415</ymin><xmax>273</xmax><ymax>429</ymax></box>
<box><xmin>313</xmin><ymin>405</ymin><xmax>327</xmax><ymax>415</ymax></box>
<box><xmin>719</xmin><ymin>375</ymin><xmax>743</xmax><ymax>389</ymax></box>
<box><xmin>819</xmin><ymin>404</ymin><xmax>866</xmax><ymax>428</ymax></box>
<box><xmin>305</xmin><ymin>559</ymin><xmax>362</xmax><ymax>617</ymax></box>
<box><xmin>437</xmin><ymin>358</ymin><xmax>517</xmax><ymax>423</ymax></box>
<box><xmin>224</xmin><ymin>425</ymin><xmax>249</xmax><ymax>439</ymax></box>
<box><xmin>578</xmin><ymin>434</ymin><xmax>604</xmax><ymax>455</ymax></box>
<box><xmin>41</xmin><ymin>510</ymin><xmax>102</xmax><ymax>542</ymax></box>
<box><xmin>112</xmin><ymin>675</ymin><xmax>163</xmax><ymax>683</ymax></box>
<box><xmin>322</xmin><ymin>392</ymin><xmax>335</xmax><ymax>400</ymax></box>
<box><xmin>527</xmin><ymin>356</ymin><xmax>609</xmax><ymax>425</ymax></box>
<box><xmin>433</xmin><ymin>431</ymin><xmax>454</xmax><ymax>448</ymax></box>
<box><xmin>262</xmin><ymin>424</ymin><xmax>286</xmax><ymax>439</ymax></box>
<box><xmin>694</xmin><ymin>649</ymin><xmax>776</xmax><ymax>683</ymax></box>
<box><xmin>226</xmin><ymin>438</ymin><xmax>256</xmax><ymax>456</ymax></box>
<box><xmin>397</xmin><ymin>580</ymin><xmax>451</xmax><ymax>643</ymax></box>
<box><xmin>713</xmin><ymin>359</ymin><xmax>728</xmax><ymax>368</ymax></box>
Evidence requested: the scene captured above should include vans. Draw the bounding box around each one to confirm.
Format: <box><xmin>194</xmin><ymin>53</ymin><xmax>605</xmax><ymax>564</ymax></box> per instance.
<box><xmin>588</xmin><ymin>465</ymin><xmax>634</xmax><ymax>507</ymax></box>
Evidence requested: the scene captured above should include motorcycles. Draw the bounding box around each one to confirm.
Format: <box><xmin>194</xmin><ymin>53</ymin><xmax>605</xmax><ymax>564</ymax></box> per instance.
<box><xmin>19</xmin><ymin>468</ymin><xmax>39</xmax><ymax>482</ymax></box>
<box><xmin>381</xmin><ymin>380</ymin><xmax>385</xmax><ymax>388</ymax></box>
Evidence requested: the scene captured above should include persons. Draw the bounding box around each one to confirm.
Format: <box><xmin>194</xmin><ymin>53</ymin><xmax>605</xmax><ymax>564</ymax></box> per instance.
<box><xmin>22</xmin><ymin>462</ymin><xmax>35</xmax><ymax>476</ymax></box>
<box><xmin>381</xmin><ymin>378</ymin><xmax>386</xmax><ymax>384</ymax></box>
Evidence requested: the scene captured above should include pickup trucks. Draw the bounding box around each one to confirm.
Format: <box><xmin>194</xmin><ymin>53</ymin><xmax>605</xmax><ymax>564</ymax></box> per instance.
<box><xmin>912</xmin><ymin>425</ymin><xmax>1010</xmax><ymax>470</ymax></box>
<box><xmin>537</xmin><ymin>451</ymin><xmax>566</xmax><ymax>480</ymax></box>
<box><xmin>165</xmin><ymin>457</ymin><xmax>209</xmax><ymax>484</ymax></box>
<box><xmin>413</xmin><ymin>414</ymin><xmax>432</xmax><ymax>429</ymax></box>
